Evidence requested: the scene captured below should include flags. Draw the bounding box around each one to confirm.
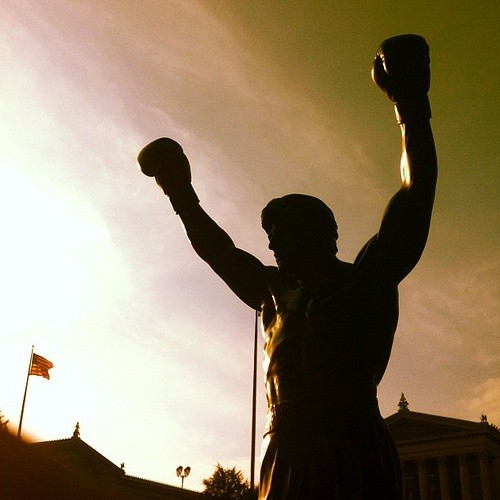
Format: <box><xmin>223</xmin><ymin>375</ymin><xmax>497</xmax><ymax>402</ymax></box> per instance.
<box><xmin>29</xmin><ymin>353</ymin><xmax>53</xmax><ymax>380</ymax></box>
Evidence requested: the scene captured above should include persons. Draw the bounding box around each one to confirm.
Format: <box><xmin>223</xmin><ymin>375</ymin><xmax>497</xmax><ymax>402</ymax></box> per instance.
<box><xmin>140</xmin><ymin>36</ymin><xmax>440</xmax><ymax>500</ymax></box>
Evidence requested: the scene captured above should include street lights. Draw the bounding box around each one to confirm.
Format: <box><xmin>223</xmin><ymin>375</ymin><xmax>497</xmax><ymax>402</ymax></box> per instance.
<box><xmin>176</xmin><ymin>464</ymin><xmax>191</xmax><ymax>489</ymax></box>
